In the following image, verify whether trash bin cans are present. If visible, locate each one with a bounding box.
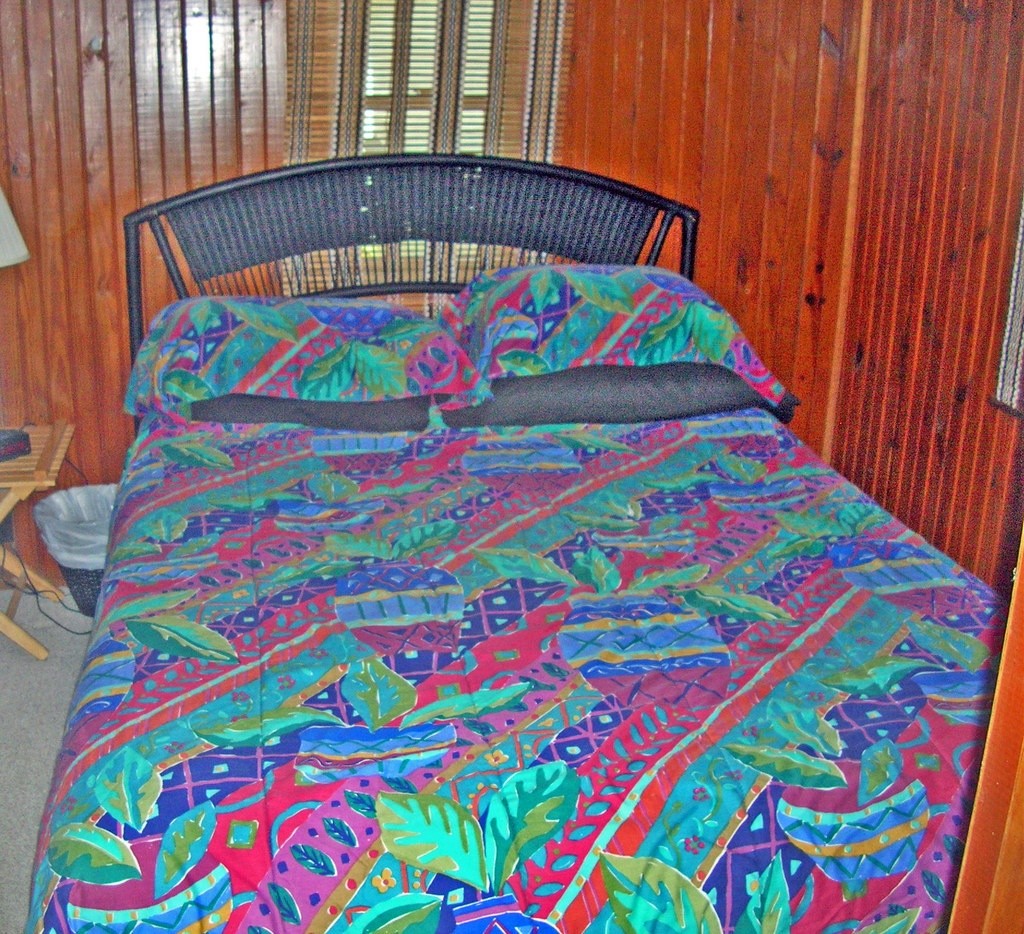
[34,484,119,616]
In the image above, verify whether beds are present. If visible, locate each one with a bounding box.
[25,152,1007,934]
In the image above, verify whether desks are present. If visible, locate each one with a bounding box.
[0,425,79,662]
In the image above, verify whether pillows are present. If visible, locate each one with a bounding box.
[121,294,493,417]
[439,267,798,421]
